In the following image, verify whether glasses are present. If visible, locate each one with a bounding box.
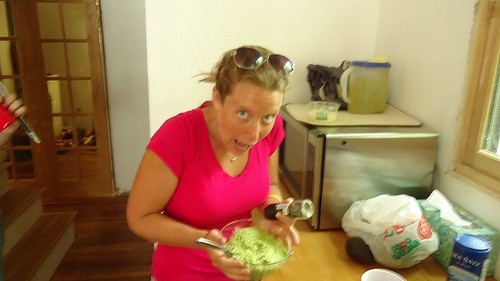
[230,47,295,78]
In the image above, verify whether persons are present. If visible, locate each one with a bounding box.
[0,90,26,146]
[127,46,299,281]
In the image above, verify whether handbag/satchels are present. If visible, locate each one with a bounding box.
[340,193,440,270]
[305,60,350,112]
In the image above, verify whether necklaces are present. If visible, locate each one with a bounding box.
[231,157,236,161]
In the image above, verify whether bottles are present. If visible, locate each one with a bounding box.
[258,198,314,221]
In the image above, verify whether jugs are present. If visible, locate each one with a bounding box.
[339,60,392,113]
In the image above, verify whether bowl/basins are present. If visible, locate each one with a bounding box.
[307,101,340,122]
[220,219,296,279]
[361,267,406,281]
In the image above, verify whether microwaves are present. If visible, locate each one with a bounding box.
[279,103,438,230]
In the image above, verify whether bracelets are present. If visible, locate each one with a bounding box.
[267,194,283,202]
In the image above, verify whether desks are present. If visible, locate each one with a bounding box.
[251,187,449,281]
